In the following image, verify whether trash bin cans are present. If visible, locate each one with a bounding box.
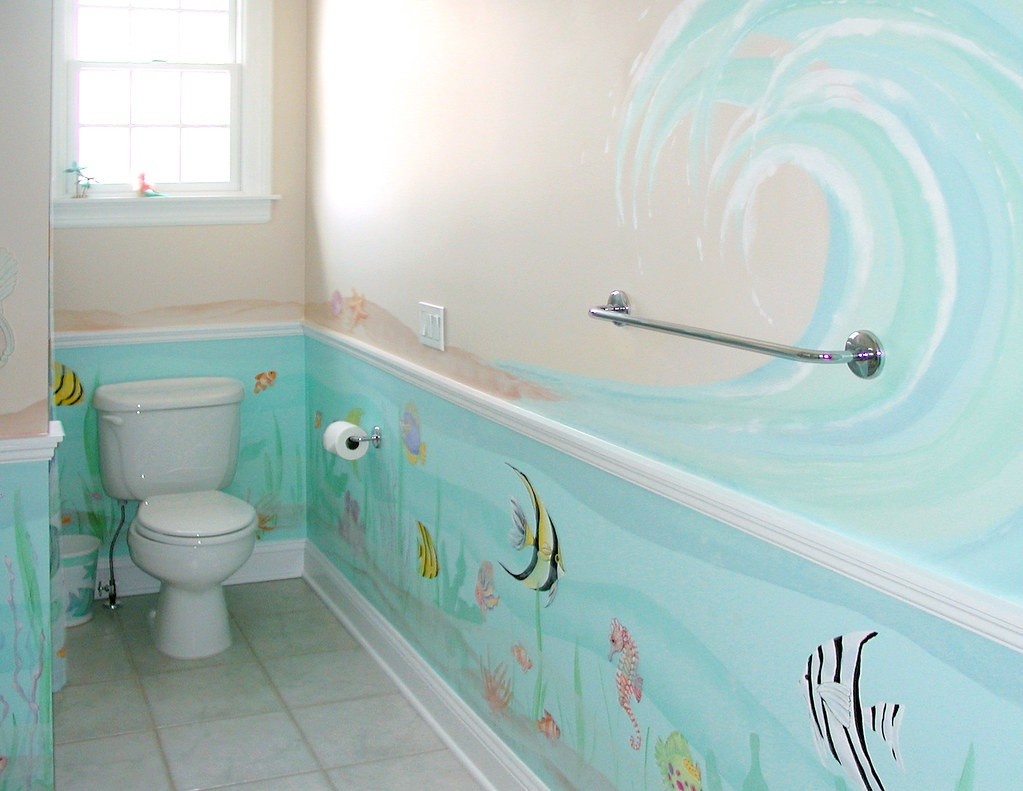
[59,534,101,628]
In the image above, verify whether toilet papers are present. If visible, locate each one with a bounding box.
[322,420,370,461]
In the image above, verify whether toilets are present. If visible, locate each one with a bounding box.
[91,374,262,662]
